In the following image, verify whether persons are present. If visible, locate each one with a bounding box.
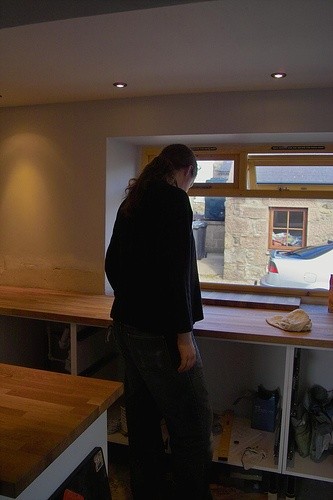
[105,144,214,500]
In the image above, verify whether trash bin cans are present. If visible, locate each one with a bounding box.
[191,220,208,261]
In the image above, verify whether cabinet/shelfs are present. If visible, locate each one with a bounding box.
[0,284,333,483]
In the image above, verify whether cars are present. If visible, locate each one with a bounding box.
[260,241,333,291]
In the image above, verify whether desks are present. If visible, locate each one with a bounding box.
[0,362,125,498]
